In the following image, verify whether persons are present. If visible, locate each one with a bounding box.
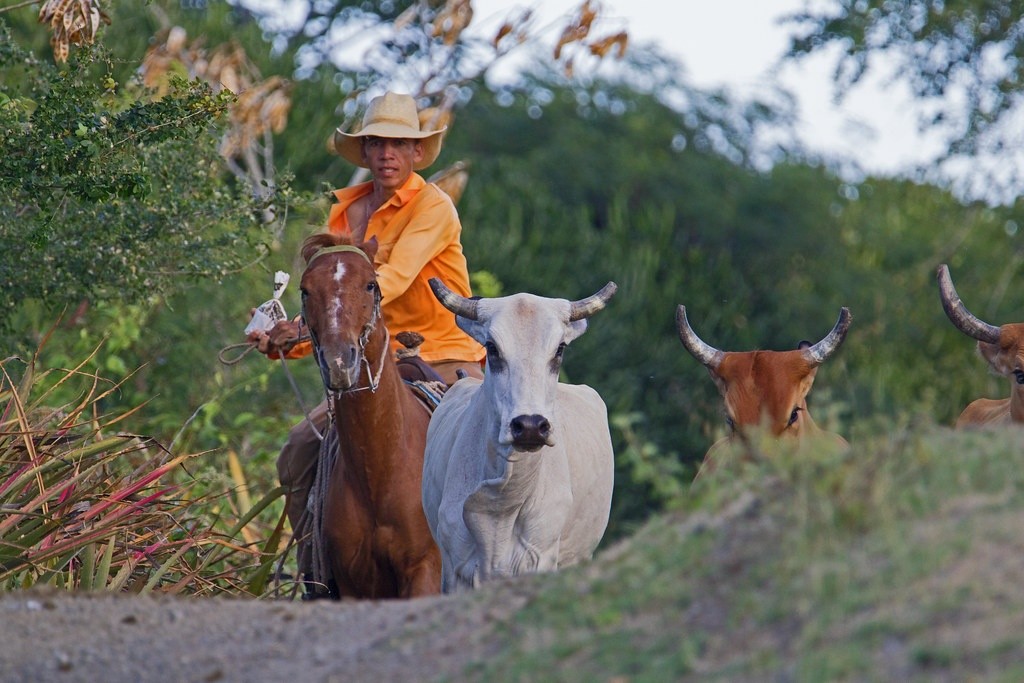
[240,90,498,603]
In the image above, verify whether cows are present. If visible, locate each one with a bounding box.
[936,264,1024,432]
[423,275,616,597]
[673,301,853,503]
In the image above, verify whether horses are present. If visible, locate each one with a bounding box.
[296,231,486,605]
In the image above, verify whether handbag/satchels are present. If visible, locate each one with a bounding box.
[244,270,291,335]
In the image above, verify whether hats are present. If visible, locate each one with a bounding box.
[335,90,444,171]
